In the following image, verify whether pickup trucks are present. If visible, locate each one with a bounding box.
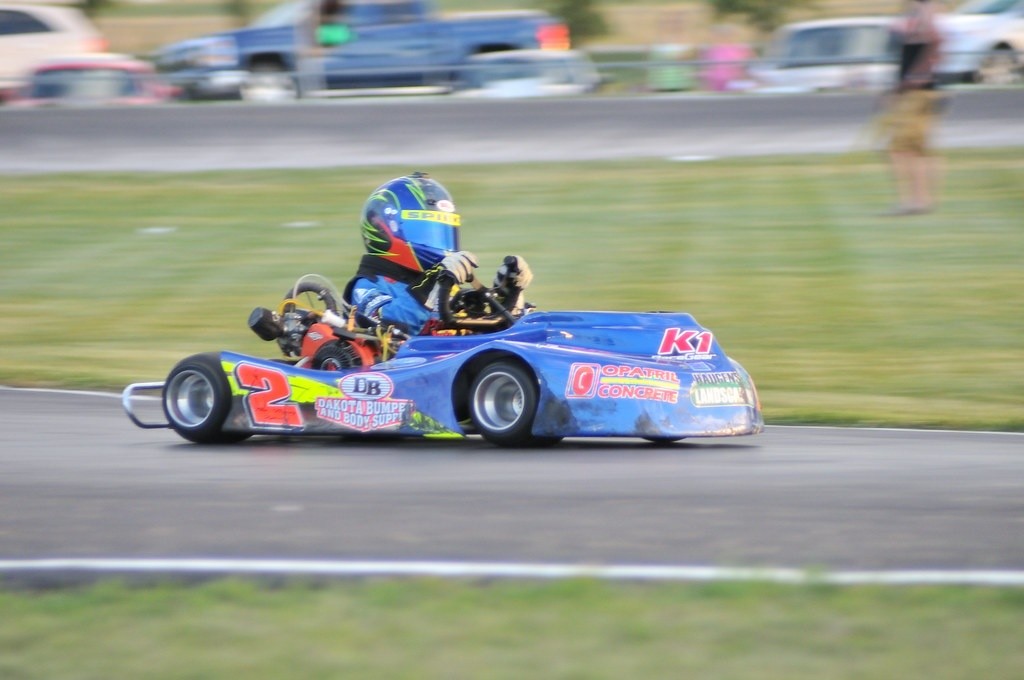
[151,0,573,100]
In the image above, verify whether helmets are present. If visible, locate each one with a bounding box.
[361,171,460,273]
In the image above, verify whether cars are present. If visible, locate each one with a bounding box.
[0,55,176,110]
[742,17,908,95]
[436,51,620,101]
[926,0,1024,88]
[0,1,107,90]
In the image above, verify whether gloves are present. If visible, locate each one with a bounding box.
[424,250,479,311]
[494,255,532,309]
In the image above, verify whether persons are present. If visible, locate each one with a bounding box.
[870,1,950,213]
[342,170,534,337]
